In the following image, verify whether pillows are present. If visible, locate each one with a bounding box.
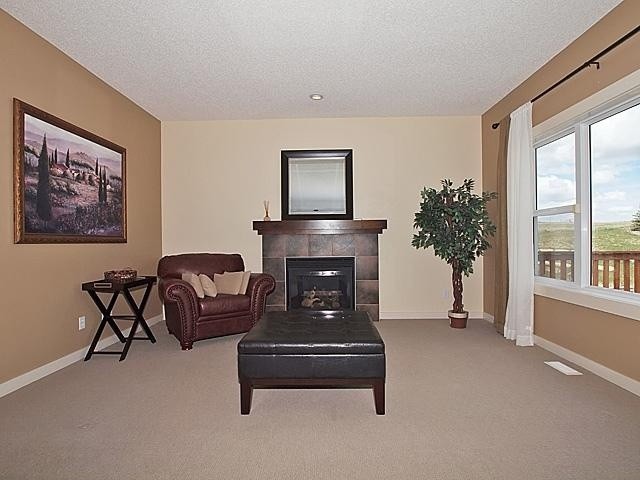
[181,270,251,298]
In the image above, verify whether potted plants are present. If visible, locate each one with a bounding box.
[412,177,498,330]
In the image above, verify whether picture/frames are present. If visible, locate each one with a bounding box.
[13,98,127,244]
[280,149,353,218]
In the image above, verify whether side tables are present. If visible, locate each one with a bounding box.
[81,275,157,361]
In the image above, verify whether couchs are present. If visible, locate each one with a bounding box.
[159,253,277,350]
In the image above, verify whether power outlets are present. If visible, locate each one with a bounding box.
[79,316,86,330]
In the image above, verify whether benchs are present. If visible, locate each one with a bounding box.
[236,310,386,415]
